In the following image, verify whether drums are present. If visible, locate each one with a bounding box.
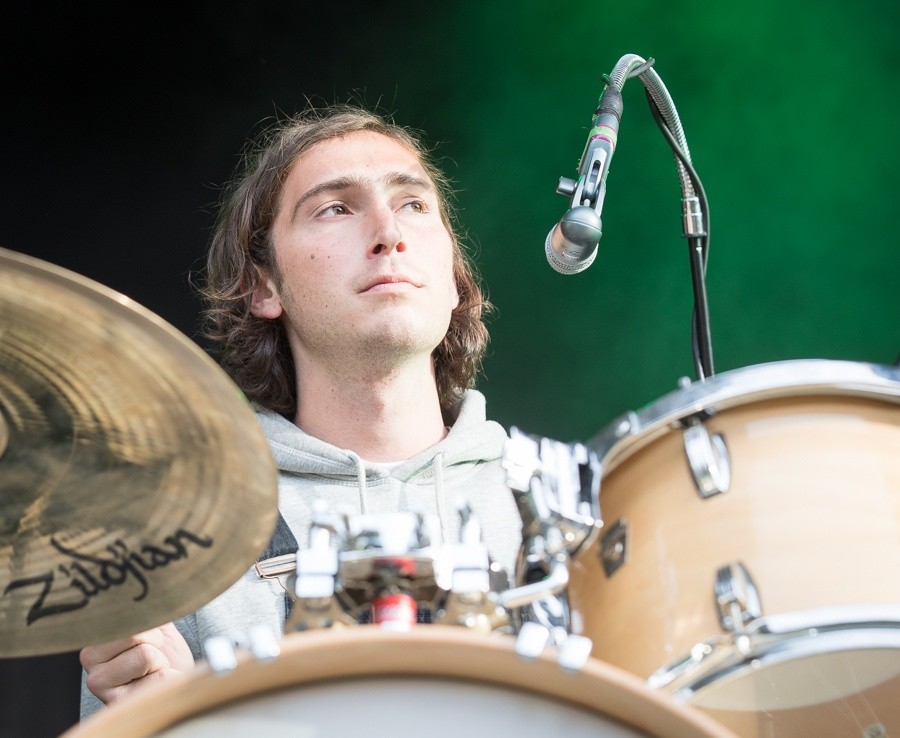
[55,620,739,738]
[514,359,900,738]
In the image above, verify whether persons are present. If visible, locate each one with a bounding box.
[79,108,638,738]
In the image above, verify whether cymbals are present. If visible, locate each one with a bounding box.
[2,247,281,659]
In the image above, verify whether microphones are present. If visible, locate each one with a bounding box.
[545,211,604,275]
